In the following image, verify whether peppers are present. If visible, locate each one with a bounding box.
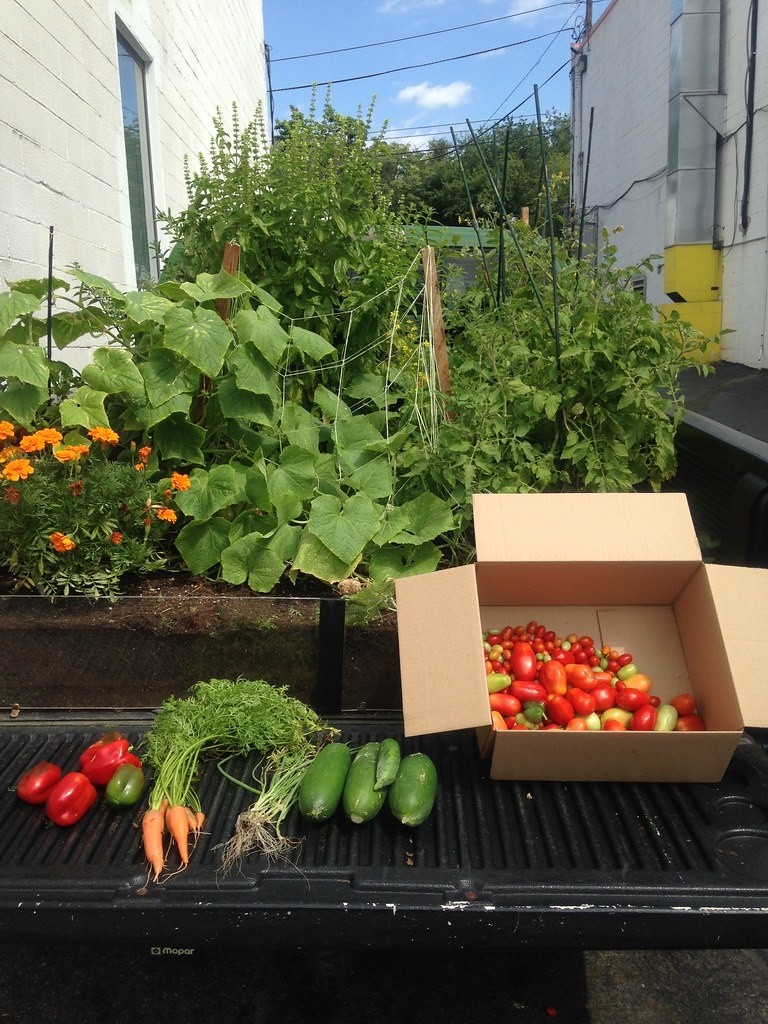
[16,731,145,827]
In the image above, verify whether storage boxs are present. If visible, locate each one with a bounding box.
[393,491,768,784]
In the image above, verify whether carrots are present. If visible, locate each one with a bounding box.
[135,674,341,894]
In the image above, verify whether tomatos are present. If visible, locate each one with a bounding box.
[482,620,705,731]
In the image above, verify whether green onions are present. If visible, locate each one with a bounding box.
[209,738,365,891]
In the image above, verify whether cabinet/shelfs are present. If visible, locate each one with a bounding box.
[0,721,768,1024]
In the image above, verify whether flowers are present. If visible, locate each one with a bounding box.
[0,416,192,603]
